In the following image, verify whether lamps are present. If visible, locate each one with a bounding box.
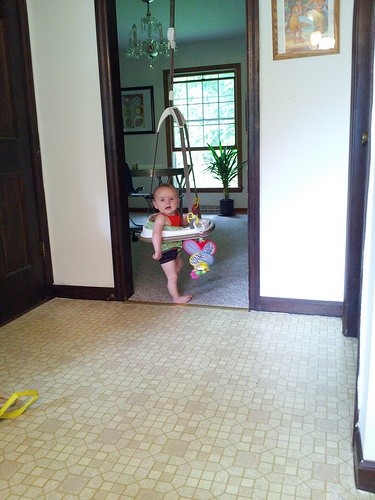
[124,0,178,70]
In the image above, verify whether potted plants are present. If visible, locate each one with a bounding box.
[203,141,247,217]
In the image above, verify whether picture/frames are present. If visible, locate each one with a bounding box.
[120,85,157,135]
[269,1,341,62]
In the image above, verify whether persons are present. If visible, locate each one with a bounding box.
[150,186,193,304]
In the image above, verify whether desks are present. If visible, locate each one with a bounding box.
[128,161,193,221]
